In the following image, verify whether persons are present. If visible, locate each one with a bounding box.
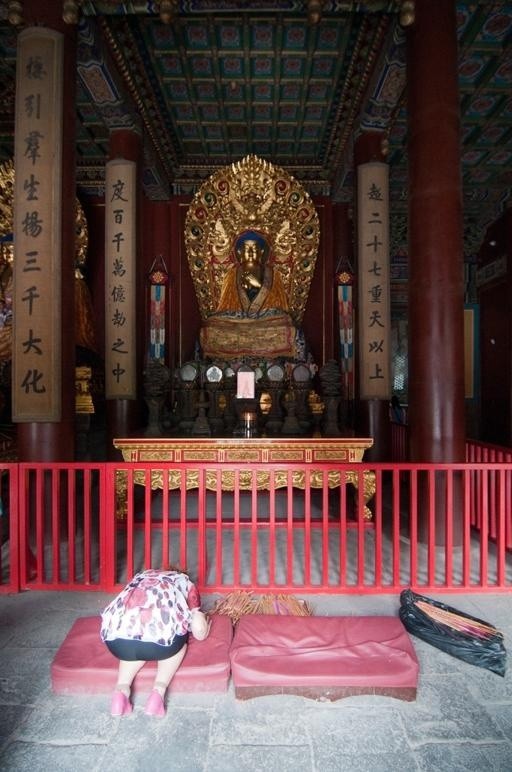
[214,232,288,316]
[101,567,212,717]
[0,234,14,327]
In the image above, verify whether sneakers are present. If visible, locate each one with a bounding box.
[111,692,132,716]
[143,689,165,717]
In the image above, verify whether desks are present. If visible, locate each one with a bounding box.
[112,437,379,522]
